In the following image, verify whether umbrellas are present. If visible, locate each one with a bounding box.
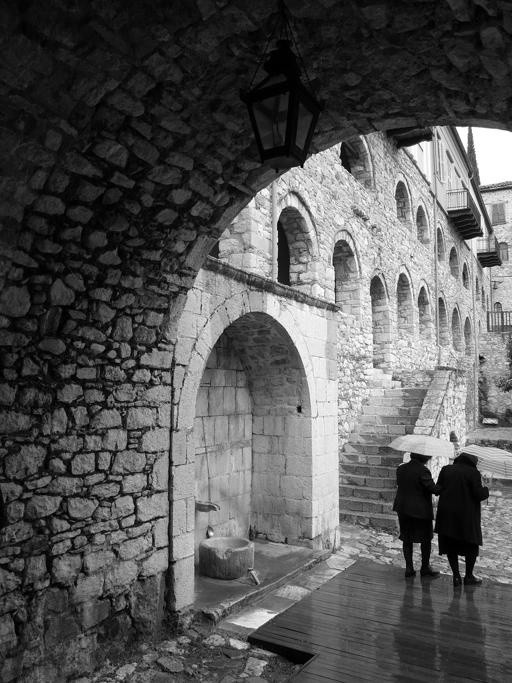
[454,443,512,481]
[384,432,457,460]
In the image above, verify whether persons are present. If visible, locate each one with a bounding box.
[391,452,439,580]
[433,452,491,588]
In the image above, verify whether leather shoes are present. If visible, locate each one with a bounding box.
[454,576,461,585]
[404,569,415,577]
[464,576,482,584]
[420,569,440,576]
